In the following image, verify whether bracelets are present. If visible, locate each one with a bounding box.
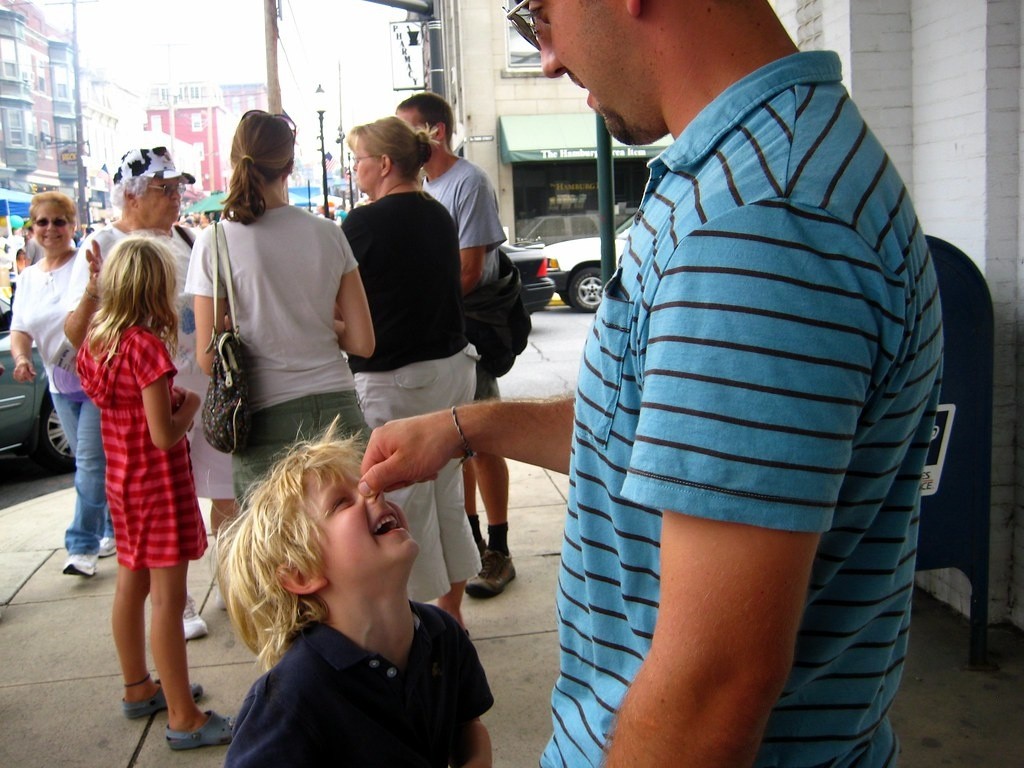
[82,286,101,304]
[12,352,33,368]
[452,406,474,471]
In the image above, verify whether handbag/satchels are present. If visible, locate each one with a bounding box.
[460,250,532,380]
[201,222,249,456]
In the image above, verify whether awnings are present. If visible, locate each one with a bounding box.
[498,114,674,164]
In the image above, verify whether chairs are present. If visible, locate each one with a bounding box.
[915,233,1000,672]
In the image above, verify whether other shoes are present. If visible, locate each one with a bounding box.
[181,598,206,640]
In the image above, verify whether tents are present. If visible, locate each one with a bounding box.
[0,189,33,284]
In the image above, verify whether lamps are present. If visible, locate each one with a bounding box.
[408,32,429,46]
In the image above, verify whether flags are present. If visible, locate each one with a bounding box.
[321,151,336,174]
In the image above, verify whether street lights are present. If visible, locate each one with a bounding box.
[314,84,332,218]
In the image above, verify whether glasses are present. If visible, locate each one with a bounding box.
[240,109,297,145]
[31,216,71,229]
[503,0,546,56]
[146,182,188,196]
[352,154,380,162]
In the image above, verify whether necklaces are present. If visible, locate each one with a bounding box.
[384,181,416,194]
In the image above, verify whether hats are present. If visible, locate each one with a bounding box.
[111,147,196,187]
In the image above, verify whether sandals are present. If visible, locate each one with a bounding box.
[164,708,237,749]
[119,678,203,720]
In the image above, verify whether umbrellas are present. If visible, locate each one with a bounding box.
[182,192,310,213]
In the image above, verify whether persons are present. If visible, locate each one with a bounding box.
[224,414,495,768]
[359,0,941,768]
[7,93,516,752]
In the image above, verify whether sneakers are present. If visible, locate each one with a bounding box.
[464,549,515,596]
[98,537,117,557]
[62,553,98,576]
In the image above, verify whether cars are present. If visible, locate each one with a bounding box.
[0,328,77,477]
[543,213,636,313]
[499,243,557,313]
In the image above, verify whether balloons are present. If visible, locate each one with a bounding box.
[9,215,24,228]
